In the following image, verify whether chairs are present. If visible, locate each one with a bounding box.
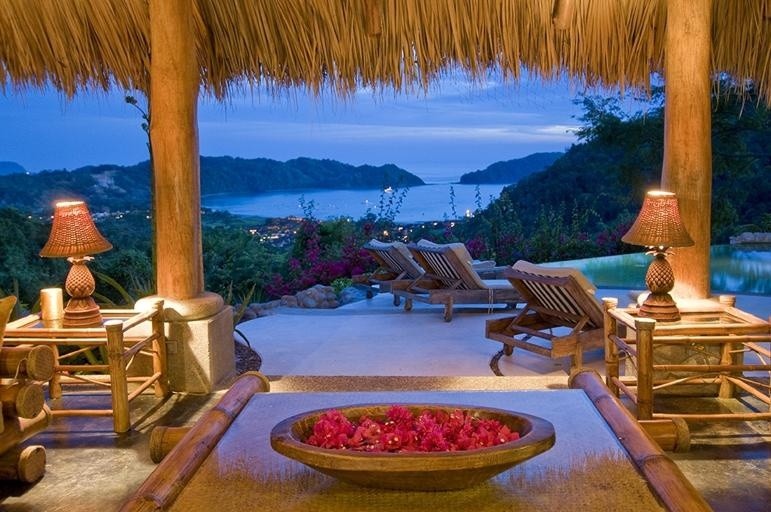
[484,258,627,376]
[362,239,528,323]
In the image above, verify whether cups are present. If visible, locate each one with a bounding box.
[40,287,64,321]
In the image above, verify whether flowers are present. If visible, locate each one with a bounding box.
[301,402,521,456]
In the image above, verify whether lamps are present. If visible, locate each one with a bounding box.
[40,201,114,328]
[621,189,696,322]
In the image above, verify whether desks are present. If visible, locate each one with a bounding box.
[3,298,172,434]
[120,369,712,511]
[602,295,771,422]
